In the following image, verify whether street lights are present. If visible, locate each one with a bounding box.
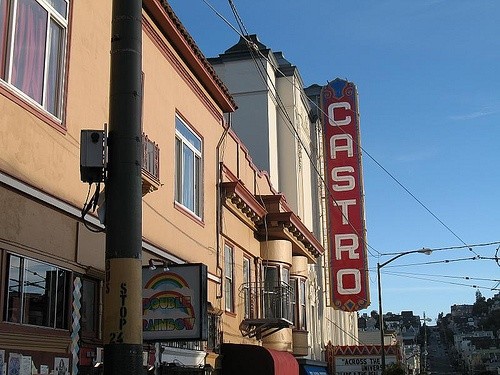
[377,249,432,375]
